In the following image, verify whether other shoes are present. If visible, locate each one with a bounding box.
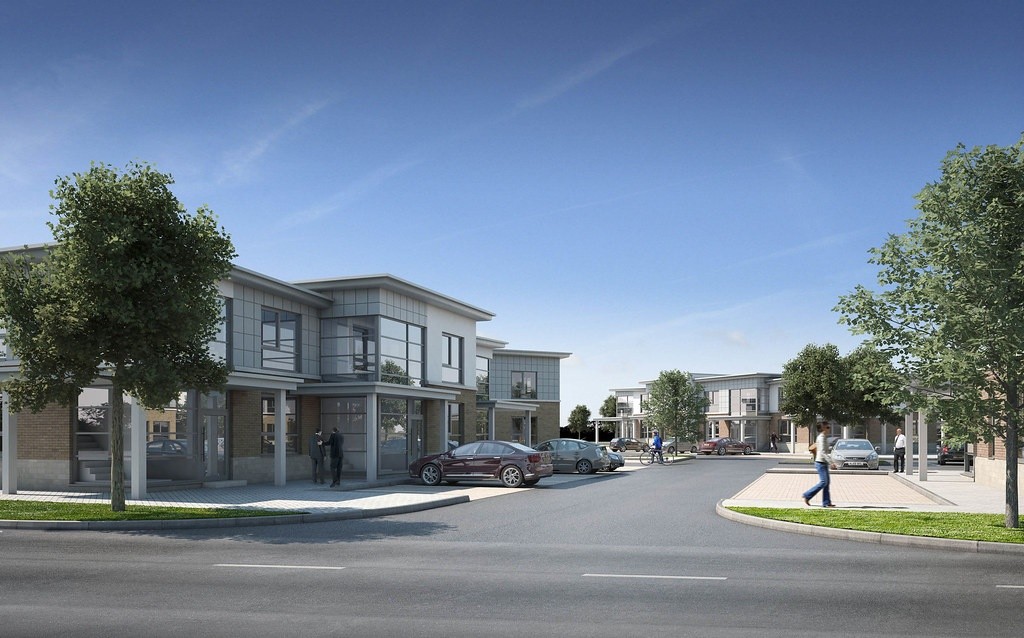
[894,470,898,473]
[900,470,904,472]
[801,495,810,506]
[823,504,836,507]
[330,482,340,487]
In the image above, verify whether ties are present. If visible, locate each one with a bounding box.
[894,434,900,446]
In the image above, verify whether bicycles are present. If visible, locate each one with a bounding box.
[640,448,674,465]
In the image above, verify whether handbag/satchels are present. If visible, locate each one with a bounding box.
[809,443,816,460]
[771,442,774,447]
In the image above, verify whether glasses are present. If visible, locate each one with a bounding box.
[823,426,830,429]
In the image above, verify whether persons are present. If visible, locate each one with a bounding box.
[309,430,327,484]
[768,432,781,452]
[648,432,664,464]
[317,427,344,487]
[892,428,906,472]
[801,421,836,508]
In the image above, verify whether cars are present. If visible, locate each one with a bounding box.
[606,451,624,471]
[408,441,554,488]
[661,436,699,454]
[218,438,274,452]
[144,439,208,457]
[936,444,973,466]
[531,438,610,475]
[829,439,881,470]
[381,436,459,452]
[825,436,842,454]
[609,437,650,453]
[700,437,754,455]
